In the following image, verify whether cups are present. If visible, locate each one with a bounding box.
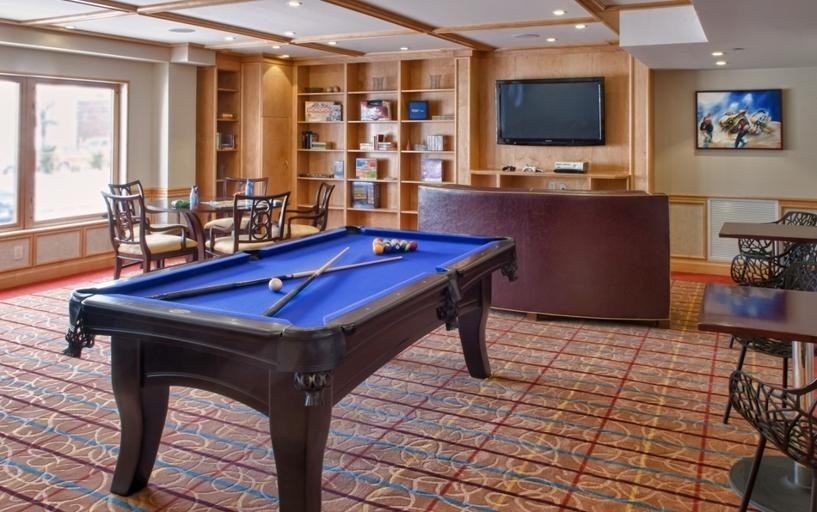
[372,77,384,91]
[429,75,441,90]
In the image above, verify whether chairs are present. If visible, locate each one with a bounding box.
[696,212,816,511]
[102,174,333,278]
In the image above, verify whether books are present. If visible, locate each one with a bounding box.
[301,135,448,150]
[218,113,236,150]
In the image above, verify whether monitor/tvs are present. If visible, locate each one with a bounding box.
[495,76,606,148]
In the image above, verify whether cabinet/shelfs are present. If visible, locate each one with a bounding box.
[242,53,299,223]
[197,54,241,225]
[295,50,458,234]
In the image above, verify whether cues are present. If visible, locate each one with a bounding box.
[144,247,404,315]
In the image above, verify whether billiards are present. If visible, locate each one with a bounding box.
[270,278,282,290]
[373,238,416,254]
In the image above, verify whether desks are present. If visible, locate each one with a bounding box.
[468,169,631,190]
[413,182,672,327]
[64,225,516,512]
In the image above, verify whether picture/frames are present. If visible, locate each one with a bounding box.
[694,87,783,154]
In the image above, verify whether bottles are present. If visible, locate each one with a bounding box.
[189,186,199,209]
[245,178,253,196]
